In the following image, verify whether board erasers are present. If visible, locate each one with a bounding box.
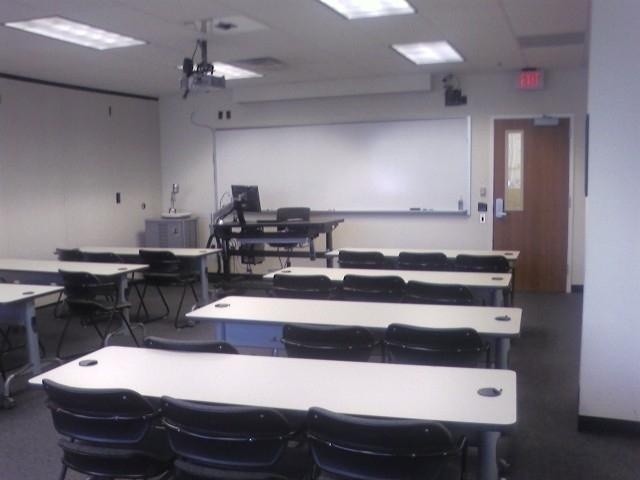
[410,208,420,210]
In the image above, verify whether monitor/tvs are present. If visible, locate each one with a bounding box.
[230,185,261,213]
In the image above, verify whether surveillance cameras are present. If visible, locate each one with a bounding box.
[441,74,455,84]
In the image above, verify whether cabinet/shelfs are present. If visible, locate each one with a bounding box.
[143,215,197,249]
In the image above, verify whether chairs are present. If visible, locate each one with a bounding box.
[40,378,164,480]
[0,248,525,406]
[152,393,296,478]
[303,403,464,479]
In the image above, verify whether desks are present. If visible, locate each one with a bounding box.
[29,345,519,477]
[209,218,345,272]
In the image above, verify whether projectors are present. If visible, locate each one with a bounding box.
[178,72,225,95]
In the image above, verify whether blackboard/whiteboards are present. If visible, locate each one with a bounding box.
[212,115,471,216]
